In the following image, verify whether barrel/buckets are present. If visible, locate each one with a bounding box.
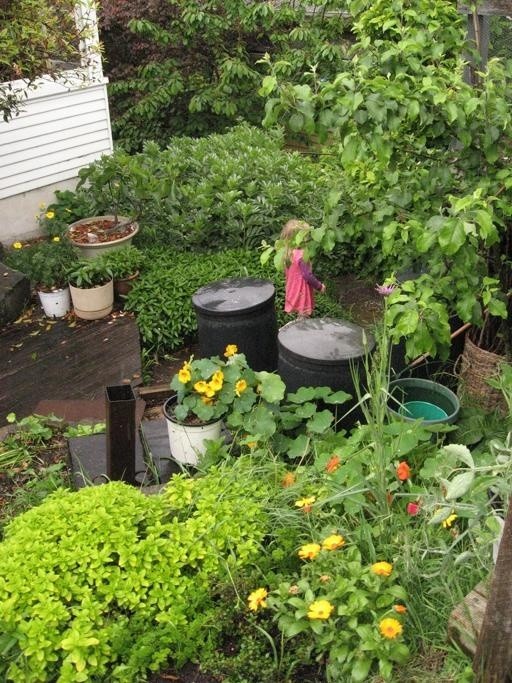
[398,401,448,420]
[69,279,114,320]
[385,378,460,426]
[37,288,72,318]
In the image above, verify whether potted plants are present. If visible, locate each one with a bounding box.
[13,172,162,320]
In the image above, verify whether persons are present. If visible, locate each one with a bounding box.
[277,219,326,319]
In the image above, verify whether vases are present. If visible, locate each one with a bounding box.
[383,378,461,447]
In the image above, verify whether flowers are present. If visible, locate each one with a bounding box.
[172,343,259,427]
